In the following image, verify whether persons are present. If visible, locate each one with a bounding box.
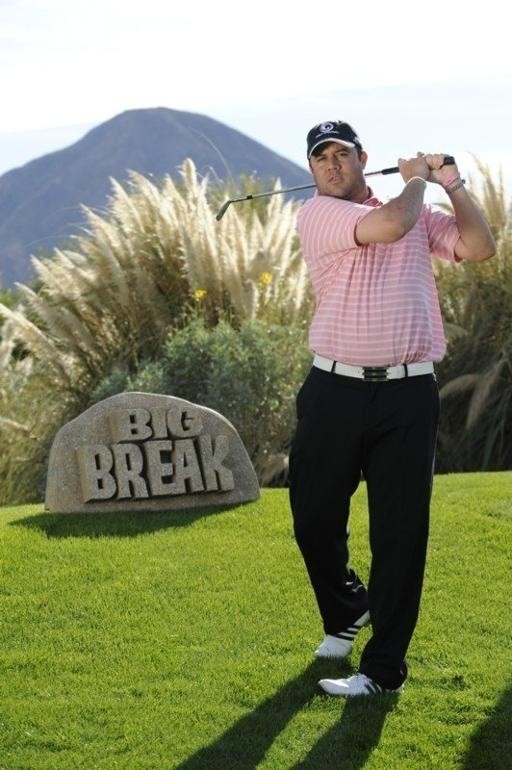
[288,118,499,699]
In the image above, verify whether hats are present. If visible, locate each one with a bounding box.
[307,120,358,159]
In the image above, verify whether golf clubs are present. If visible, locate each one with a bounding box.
[216,158,454,221]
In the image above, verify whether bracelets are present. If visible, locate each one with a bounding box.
[446,179,467,195]
[441,170,460,188]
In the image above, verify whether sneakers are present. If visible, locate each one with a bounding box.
[318,673,395,697]
[314,609,370,659]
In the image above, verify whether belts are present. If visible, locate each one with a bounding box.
[312,354,435,382]
